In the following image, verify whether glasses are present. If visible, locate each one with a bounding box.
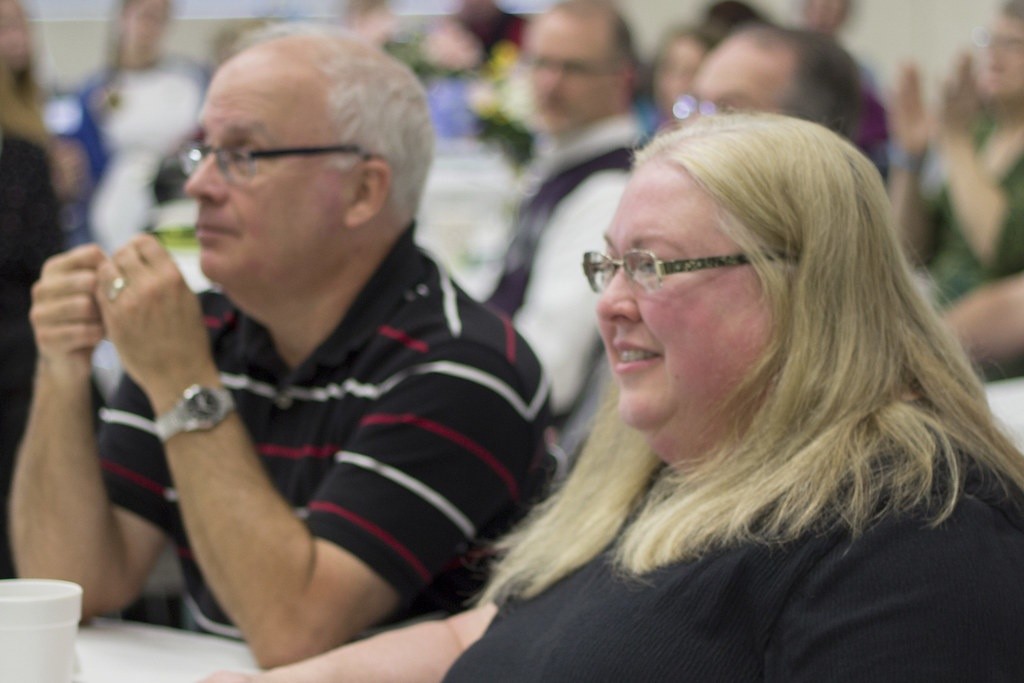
[672,93,724,119]
[177,141,371,187]
[581,251,787,293]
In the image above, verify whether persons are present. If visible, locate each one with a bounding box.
[9,32,570,672]
[0,2,1023,495]
[275,111,1023,682]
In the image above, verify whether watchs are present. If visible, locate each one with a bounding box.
[156,385,241,446]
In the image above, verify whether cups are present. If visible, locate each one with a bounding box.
[0,577,83,683]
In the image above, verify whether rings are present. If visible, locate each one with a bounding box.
[104,276,126,300]
